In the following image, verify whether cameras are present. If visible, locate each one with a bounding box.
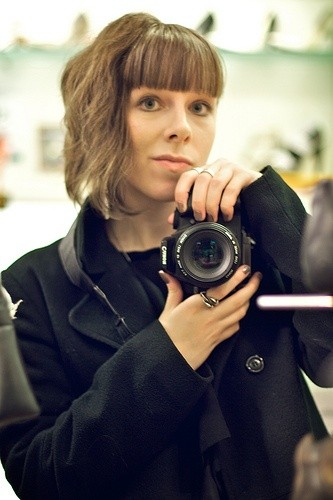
[160,194,252,296]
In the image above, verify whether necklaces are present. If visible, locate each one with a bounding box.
[106,221,130,262]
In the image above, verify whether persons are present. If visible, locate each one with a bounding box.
[0,12,333,499]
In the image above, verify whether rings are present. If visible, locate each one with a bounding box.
[200,291,219,308]
[192,167,215,177]
